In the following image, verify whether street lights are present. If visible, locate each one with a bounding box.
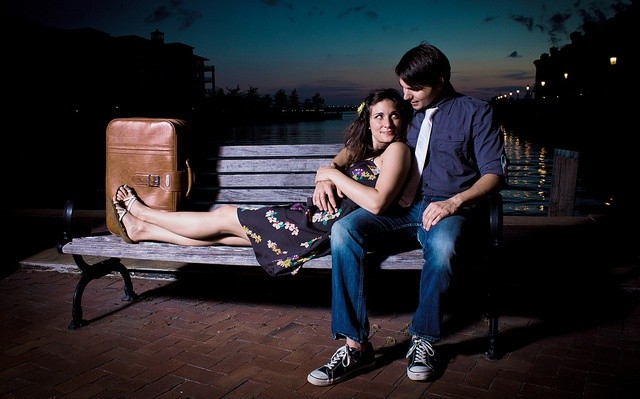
[541,81,545,95]
[526,84,530,97]
[610,56,617,91]
[509,91,512,99]
[564,73,568,95]
[517,88,519,98]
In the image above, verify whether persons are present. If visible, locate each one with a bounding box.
[307,44,511,387]
[110,88,410,275]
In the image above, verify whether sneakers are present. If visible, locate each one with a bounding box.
[406,334,436,382]
[306,341,378,386]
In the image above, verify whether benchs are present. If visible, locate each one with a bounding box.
[59,140,505,359]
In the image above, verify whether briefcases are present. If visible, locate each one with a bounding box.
[102,116,199,238]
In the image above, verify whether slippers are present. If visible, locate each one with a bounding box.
[107,196,135,244]
[114,184,139,209]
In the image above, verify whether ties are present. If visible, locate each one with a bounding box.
[396,105,439,208]
